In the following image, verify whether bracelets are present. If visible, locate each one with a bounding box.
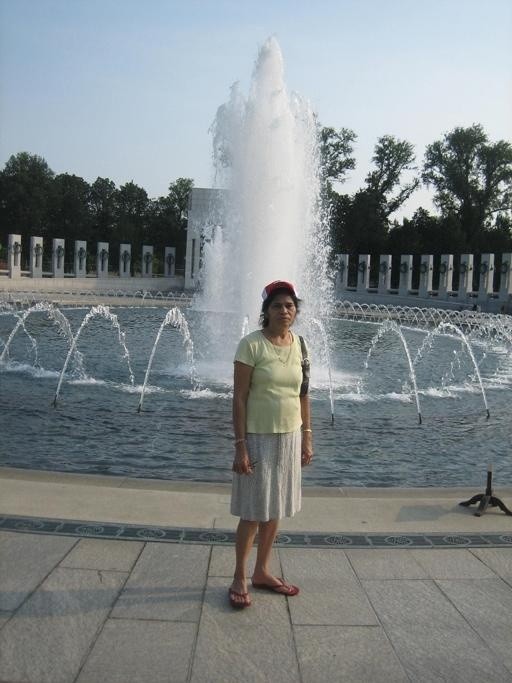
[235,439,248,446]
[304,429,312,432]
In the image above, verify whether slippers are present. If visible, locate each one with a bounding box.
[252,577,300,596]
[228,587,251,608]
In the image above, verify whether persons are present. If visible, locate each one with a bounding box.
[230,281,314,609]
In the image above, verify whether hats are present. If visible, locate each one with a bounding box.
[261,279,298,303]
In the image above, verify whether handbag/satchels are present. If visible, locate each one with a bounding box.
[299,358,311,397]
